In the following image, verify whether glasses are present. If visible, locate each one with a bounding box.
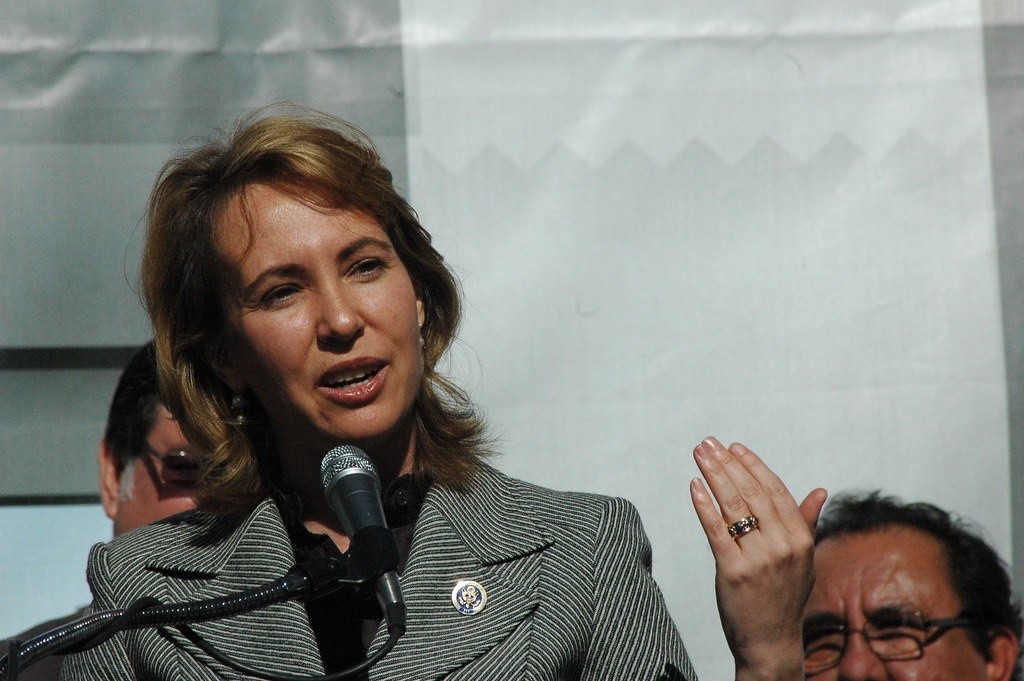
[802,609,979,669]
[123,431,200,486]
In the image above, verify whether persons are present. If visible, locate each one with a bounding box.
[60,101,827,681]
[803,494,1024,681]
[0,340,198,681]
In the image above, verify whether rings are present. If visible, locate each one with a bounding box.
[728,514,758,539]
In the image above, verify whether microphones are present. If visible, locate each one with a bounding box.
[321,445,407,635]
